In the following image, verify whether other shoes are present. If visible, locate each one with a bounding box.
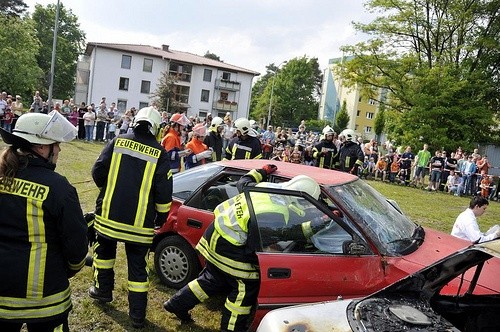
[164,299,195,322]
[129,312,144,327]
[88,286,112,301]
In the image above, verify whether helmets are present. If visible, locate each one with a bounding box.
[208,117,227,132]
[133,107,161,135]
[0,113,77,144]
[234,118,258,137]
[192,125,207,136]
[284,175,321,211]
[322,126,337,139]
[339,129,358,148]
[170,114,191,125]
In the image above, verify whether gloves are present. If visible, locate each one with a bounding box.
[323,210,341,221]
[263,164,277,175]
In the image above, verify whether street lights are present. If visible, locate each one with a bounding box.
[267,60,288,128]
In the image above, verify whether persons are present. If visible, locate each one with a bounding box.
[335,128,365,176]
[222,117,262,160]
[160,113,193,174]
[0,90,120,144]
[312,125,340,171]
[222,112,317,166]
[88,106,173,328]
[0,109,87,332]
[361,138,432,189]
[451,196,500,243]
[119,107,213,145]
[204,116,224,162]
[431,146,500,202]
[164,164,344,332]
[183,123,213,169]
[82,211,95,267]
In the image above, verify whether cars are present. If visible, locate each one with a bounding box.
[146,158,500,332]
[252,232,500,332]
